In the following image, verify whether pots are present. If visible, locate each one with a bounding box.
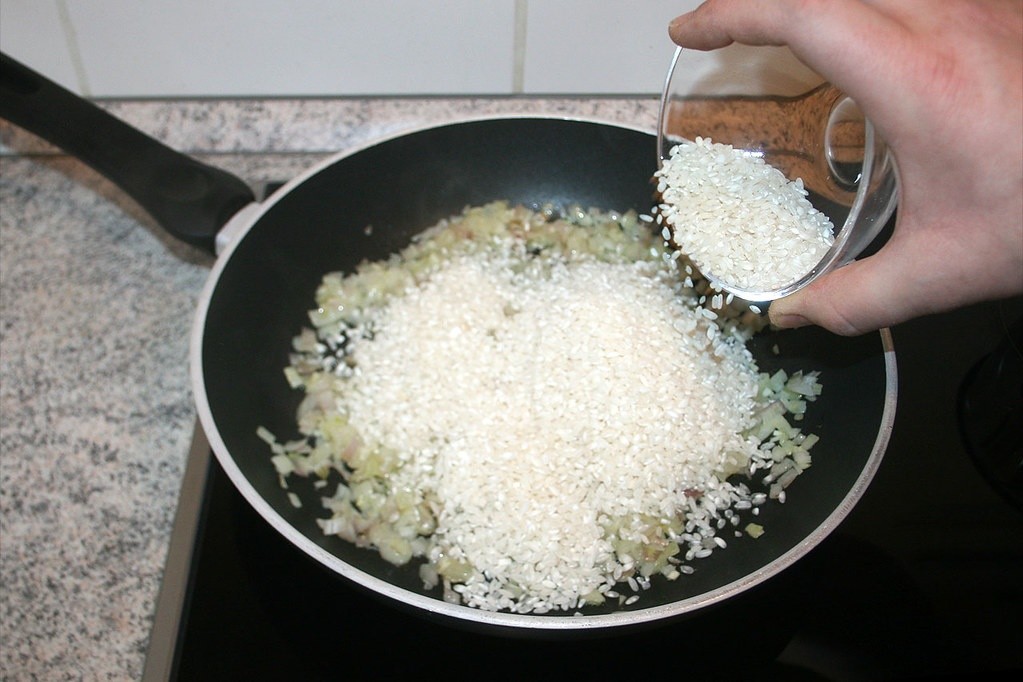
[0,46,897,633]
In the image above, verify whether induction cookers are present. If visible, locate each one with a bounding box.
[111,174,1020,682]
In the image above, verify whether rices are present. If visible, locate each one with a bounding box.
[339,137,835,615]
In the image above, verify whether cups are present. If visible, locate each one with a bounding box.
[656,31,903,302]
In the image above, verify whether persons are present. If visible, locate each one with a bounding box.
[668,1,1023,342]
